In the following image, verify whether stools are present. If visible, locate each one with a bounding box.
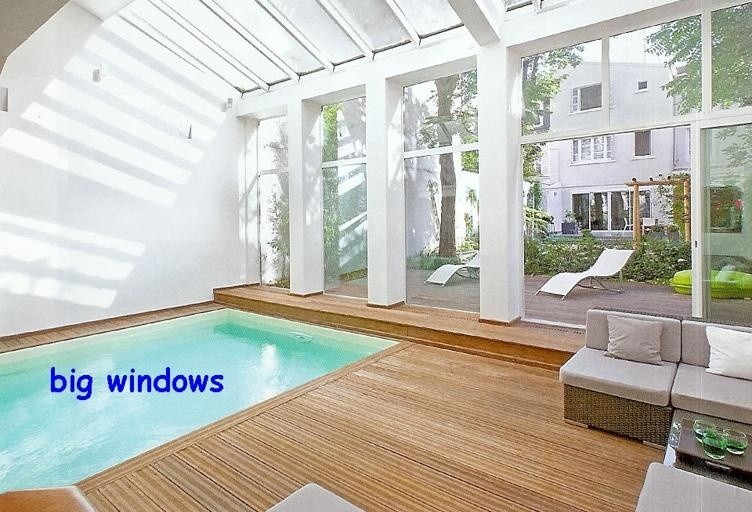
[633,462,751,512]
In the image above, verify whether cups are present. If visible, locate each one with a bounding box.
[692,419,748,460]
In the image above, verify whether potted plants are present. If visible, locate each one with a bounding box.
[560,209,578,234]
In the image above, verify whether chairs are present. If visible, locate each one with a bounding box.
[423,251,478,288]
[535,248,635,301]
[557,306,752,474]
[622,217,656,236]
